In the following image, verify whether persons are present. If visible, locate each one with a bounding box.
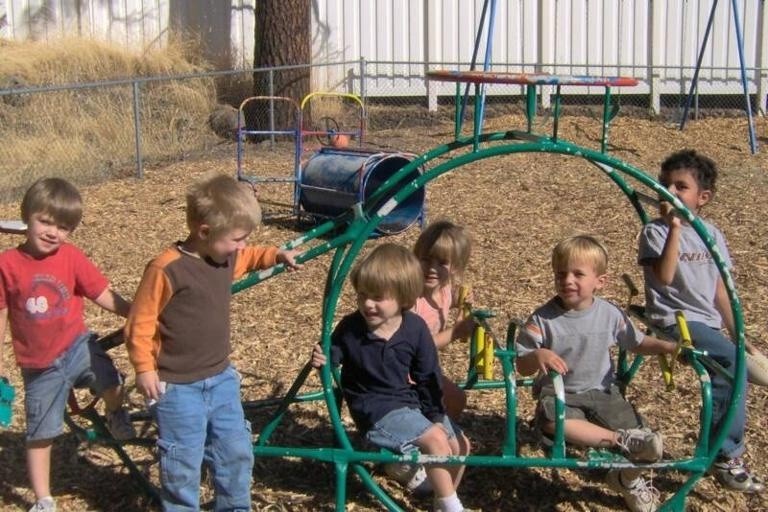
[639,147,768,490]
[312,240,478,512]
[516,233,695,511]
[2,175,133,511]
[405,219,481,426]
[123,167,304,512]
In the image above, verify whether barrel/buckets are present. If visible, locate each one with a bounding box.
[297,146,427,234]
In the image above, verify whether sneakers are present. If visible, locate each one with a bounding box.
[702,454,752,491]
[104,407,137,440]
[615,428,663,463]
[27,499,56,512]
[604,468,663,512]
